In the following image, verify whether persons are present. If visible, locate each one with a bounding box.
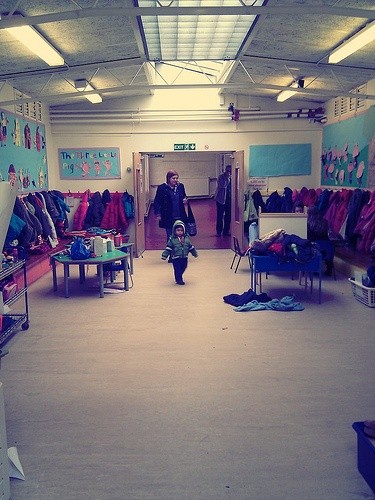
[161,220,199,285]
[154,169,189,263]
[214,165,232,237]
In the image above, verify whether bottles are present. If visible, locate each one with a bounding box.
[13,249,18,263]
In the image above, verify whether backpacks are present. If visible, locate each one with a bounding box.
[70,237,91,260]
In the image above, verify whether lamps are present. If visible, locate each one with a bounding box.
[75,80,103,105]
[2,13,65,66]
[277,79,304,103]
[326,20,375,65]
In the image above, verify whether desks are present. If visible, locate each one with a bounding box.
[113,242,134,278]
[251,252,324,304]
[54,249,128,298]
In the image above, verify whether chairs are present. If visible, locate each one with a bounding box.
[230,236,250,273]
[313,240,337,280]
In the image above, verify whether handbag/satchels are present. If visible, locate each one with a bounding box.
[3,281,17,302]
[186,219,197,237]
[249,239,266,254]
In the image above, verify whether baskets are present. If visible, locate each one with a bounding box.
[348,278,375,308]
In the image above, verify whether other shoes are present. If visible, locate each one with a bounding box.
[216,232,221,236]
[223,231,231,236]
[176,281,185,285]
